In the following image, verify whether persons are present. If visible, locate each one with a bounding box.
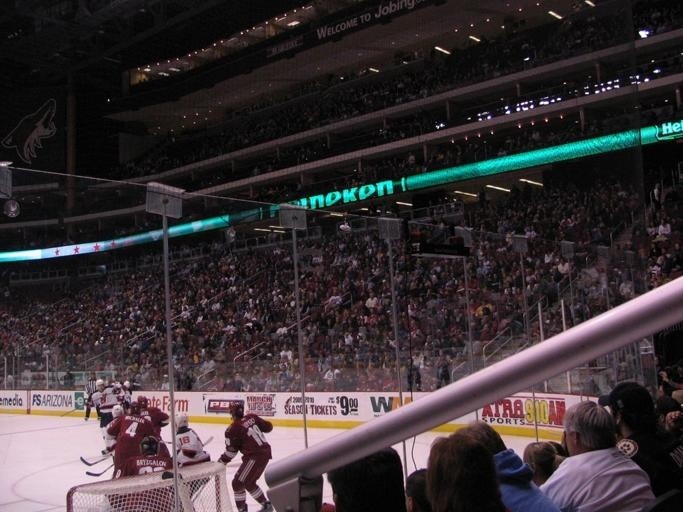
[0,3,681,390]
[326,390,683,512]
[84,389,274,511]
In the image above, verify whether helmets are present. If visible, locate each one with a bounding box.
[140,436,161,456]
[175,415,188,428]
[96,379,147,417]
[229,400,244,409]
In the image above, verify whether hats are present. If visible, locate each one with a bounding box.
[598,381,651,405]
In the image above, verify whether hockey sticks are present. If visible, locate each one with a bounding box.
[86,463,115,476]
[164,436,213,447]
[61,405,83,417]
[79,454,113,466]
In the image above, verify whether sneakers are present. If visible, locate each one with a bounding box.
[257,501,273,512]
